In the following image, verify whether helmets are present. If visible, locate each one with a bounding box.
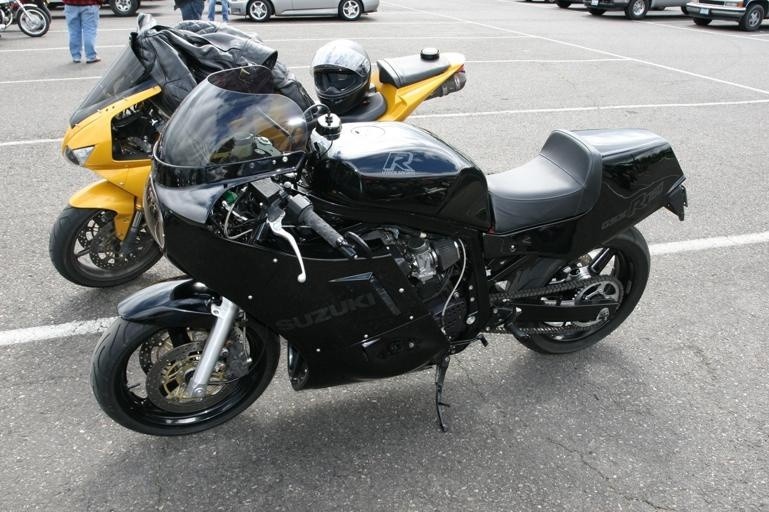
[309,40,371,114]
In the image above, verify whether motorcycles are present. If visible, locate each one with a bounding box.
[89,58,689,437]
[46,13,469,292]
[0,0,51,40]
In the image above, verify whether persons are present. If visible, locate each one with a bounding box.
[63,1,101,63]
[208,0,229,22]
[174,0,205,19]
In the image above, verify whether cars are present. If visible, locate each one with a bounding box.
[54,1,142,18]
[531,1,769,33]
[224,0,380,23]
